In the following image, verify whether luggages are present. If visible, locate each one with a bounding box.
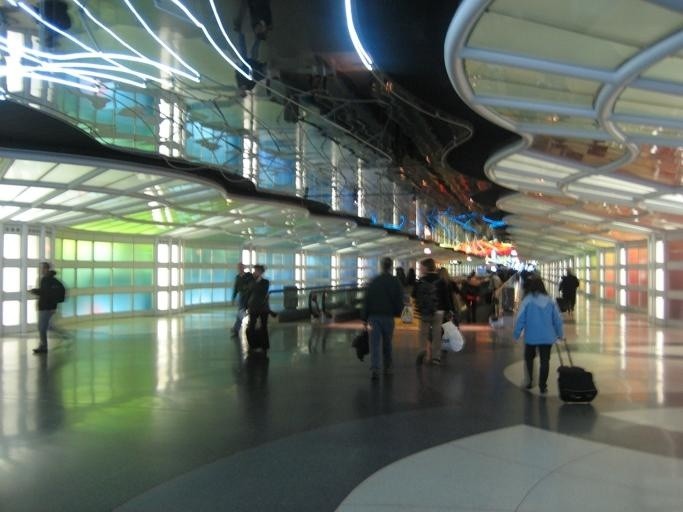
[246,329,269,348]
[555,338,598,403]
[556,290,567,312]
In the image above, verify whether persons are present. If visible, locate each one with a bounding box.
[556,267,578,315]
[360,256,406,382]
[241,263,270,350]
[393,264,512,326]
[23,260,73,353]
[509,273,565,394]
[226,261,251,337]
[409,256,446,367]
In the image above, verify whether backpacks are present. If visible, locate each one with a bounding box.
[414,279,441,316]
[52,275,65,304]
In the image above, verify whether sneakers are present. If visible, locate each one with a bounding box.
[33,348,47,353]
[416,350,427,366]
[433,359,440,365]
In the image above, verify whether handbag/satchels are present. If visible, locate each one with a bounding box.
[402,305,412,324]
[354,333,369,354]
